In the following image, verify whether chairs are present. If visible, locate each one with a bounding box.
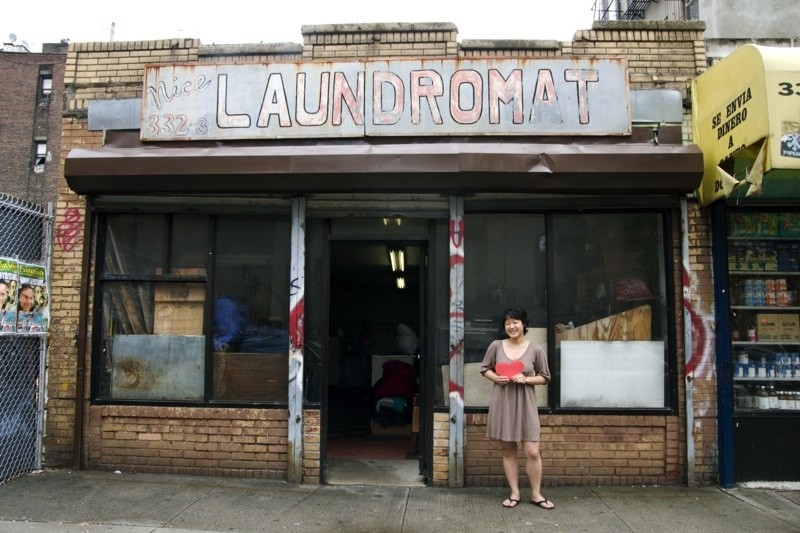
[372,355,415,439]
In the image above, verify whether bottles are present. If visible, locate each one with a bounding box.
[733,384,800,410]
[731,351,800,378]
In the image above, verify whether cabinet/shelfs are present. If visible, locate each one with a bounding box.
[728,236,800,381]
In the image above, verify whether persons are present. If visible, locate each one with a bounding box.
[0,279,47,333]
[480,306,555,510]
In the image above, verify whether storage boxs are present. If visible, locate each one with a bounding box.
[756,313,800,342]
[153,282,205,335]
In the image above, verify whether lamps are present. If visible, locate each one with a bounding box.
[387,244,405,289]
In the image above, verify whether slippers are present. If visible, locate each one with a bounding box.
[530,497,555,509]
[502,497,521,508]
